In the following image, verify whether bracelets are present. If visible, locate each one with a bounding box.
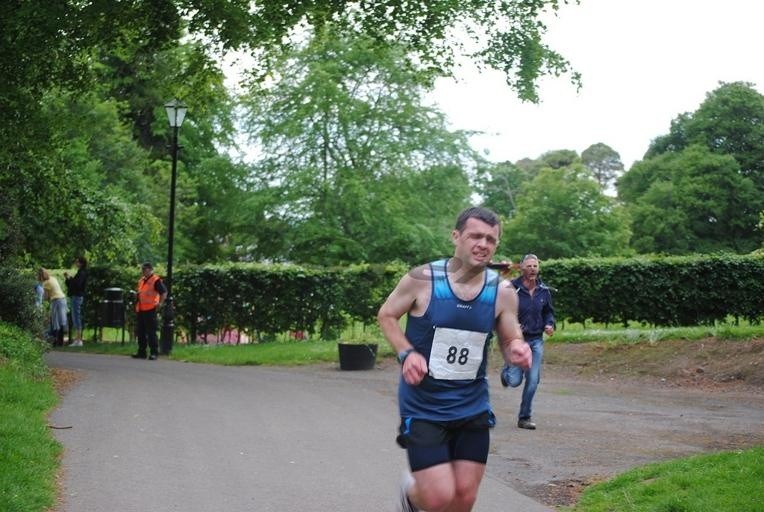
[395,347,415,365]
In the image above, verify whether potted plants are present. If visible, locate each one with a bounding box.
[337,332,378,370]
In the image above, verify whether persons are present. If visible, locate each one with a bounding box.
[61,255,87,347]
[129,263,168,361]
[500,252,555,431]
[376,204,533,511]
[36,268,68,346]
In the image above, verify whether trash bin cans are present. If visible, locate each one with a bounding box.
[98,287,125,345]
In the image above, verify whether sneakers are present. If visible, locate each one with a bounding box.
[149,355,157,360]
[400,476,419,512]
[500,370,509,387]
[131,353,147,359]
[518,419,537,431]
[68,340,83,347]
